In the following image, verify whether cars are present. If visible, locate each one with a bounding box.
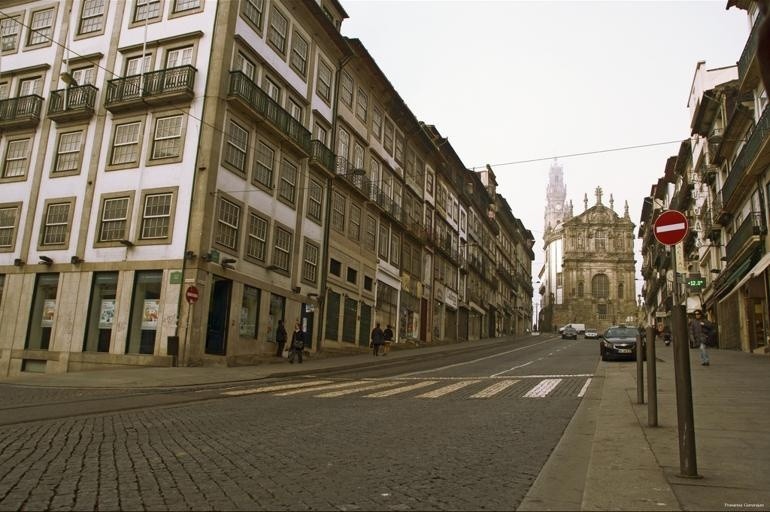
[562,327,577,340]
[584,328,599,340]
[601,324,647,362]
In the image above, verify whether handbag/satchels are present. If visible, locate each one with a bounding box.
[294,340,303,348]
[370,341,374,347]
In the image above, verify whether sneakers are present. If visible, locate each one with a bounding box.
[701,362,710,366]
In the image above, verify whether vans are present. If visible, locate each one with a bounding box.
[559,323,585,335]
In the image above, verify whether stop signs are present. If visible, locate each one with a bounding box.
[186,286,199,304]
[653,209,688,246]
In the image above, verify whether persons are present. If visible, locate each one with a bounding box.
[289,323,305,364]
[276,319,288,358]
[688,309,718,366]
[371,323,393,356]
